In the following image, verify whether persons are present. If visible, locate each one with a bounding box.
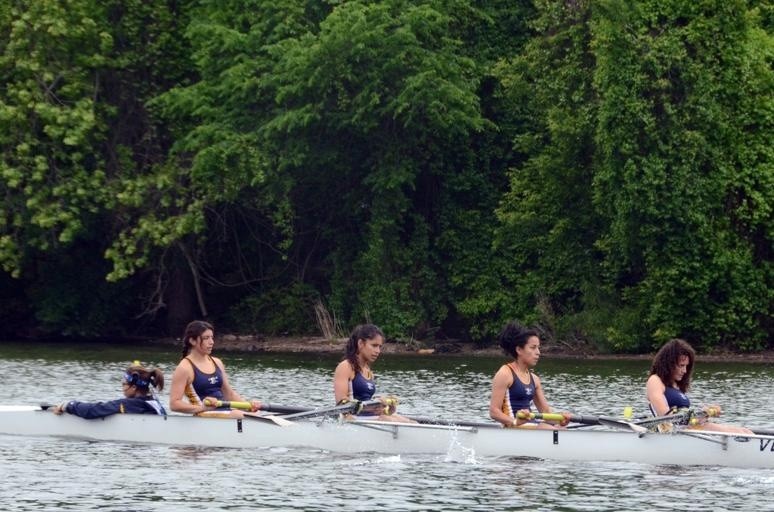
[646,338,755,435]
[488,321,571,430]
[169,321,261,418]
[334,325,419,424]
[47,365,167,420]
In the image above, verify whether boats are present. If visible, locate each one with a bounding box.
[0,399,774,469]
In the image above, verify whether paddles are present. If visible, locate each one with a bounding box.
[598,409,716,434]
[243,399,395,427]
